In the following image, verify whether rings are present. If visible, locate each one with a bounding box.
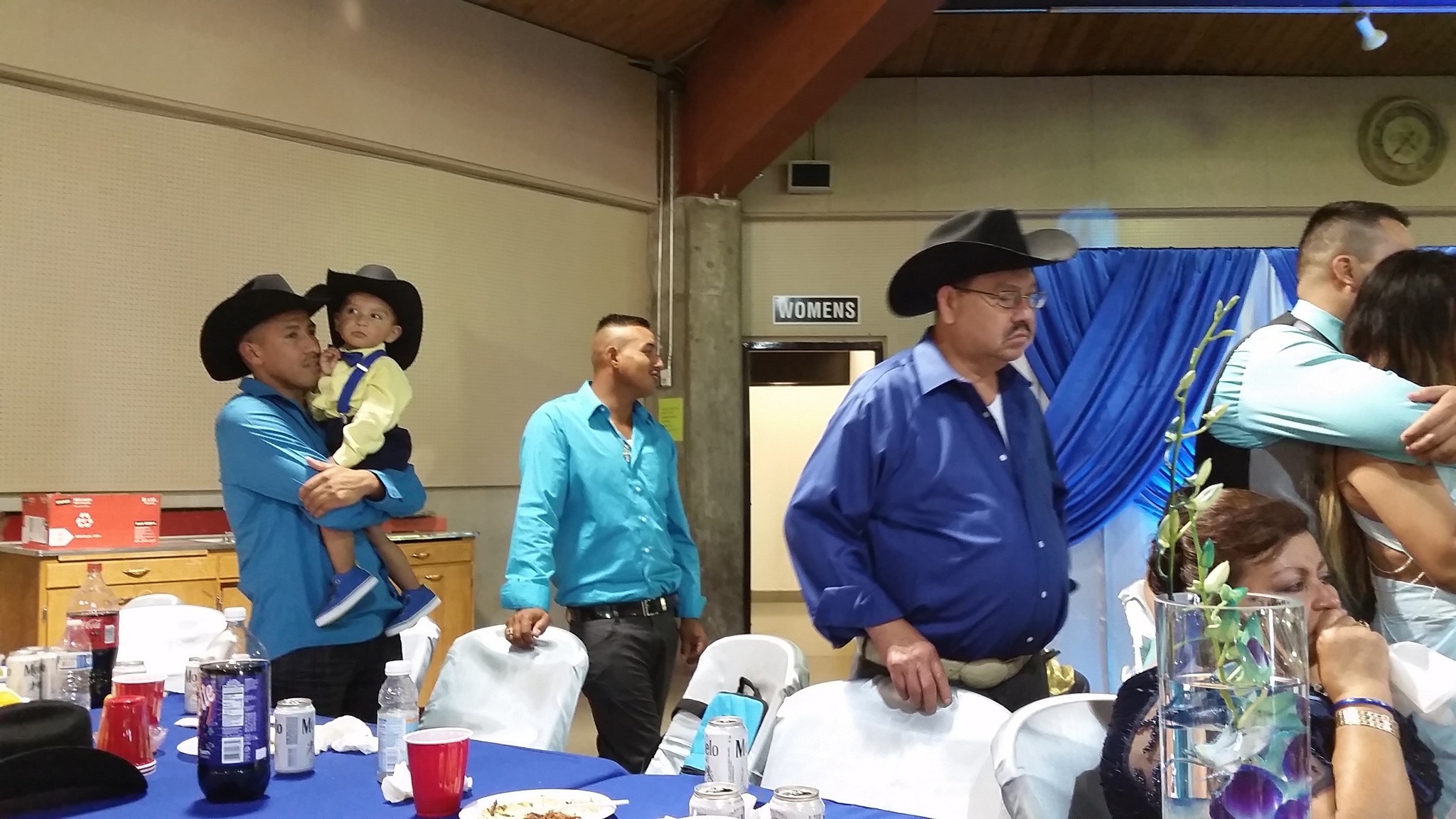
[504,628,514,635]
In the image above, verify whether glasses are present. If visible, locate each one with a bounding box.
[954,285,1049,309]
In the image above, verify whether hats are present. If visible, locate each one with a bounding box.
[323,264,423,371]
[885,207,1078,319]
[0,700,149,808]
[200,274,329,382]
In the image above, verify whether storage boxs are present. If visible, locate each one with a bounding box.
[14,484,165,551]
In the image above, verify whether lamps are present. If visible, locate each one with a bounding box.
[1354,4,1390,53]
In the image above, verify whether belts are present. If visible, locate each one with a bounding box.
[567,590,679,622]
[861,637,1035,688]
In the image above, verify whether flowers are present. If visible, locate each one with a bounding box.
[1144,299,1330,818]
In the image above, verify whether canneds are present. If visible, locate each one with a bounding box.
[5,644,56,704]
[273,696,317,774]
[186,656,208,715]
[684,720,824,818]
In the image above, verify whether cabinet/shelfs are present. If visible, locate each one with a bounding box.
[0,528,482,724]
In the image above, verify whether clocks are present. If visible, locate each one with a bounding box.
[1356,96,1450,186]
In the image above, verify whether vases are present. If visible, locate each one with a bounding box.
[1149,595,1314,819]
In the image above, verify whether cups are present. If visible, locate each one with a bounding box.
[4,648,68,702]
[403,727,473,817]
[92,668,169,776]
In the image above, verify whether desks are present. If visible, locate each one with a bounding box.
[0,651,945,817]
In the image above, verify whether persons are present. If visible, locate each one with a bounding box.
[1097,485,1444,819]
[1307,244,1455,819]
[1192,199,1454,625]
[782,208,1088,711]
[304,266,441,636]
[197,273,428,724]
[502,313,708,775]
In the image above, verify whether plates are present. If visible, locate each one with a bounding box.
[457,788,616,819]
[177,726,277,758]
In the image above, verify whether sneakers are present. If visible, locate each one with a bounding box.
[314,562,380,629]
[385,585,441,638]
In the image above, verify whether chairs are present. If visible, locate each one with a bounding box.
[412,607,1143,819]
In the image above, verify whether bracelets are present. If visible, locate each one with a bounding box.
[1331,699,1402,723]
[1334,708,1401,738]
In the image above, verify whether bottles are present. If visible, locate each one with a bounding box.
[378,658,421,786]
[67,562,122,708]
[194,607,272,805]
[55,618,93,714]
